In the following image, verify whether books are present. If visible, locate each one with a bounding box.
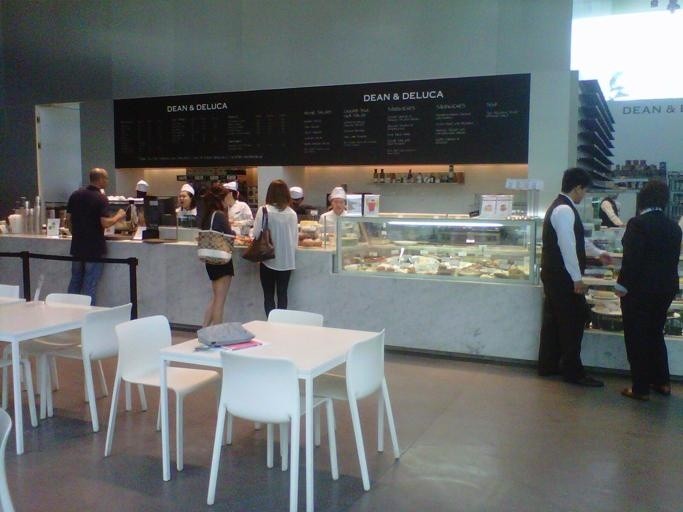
[221,340,263,351]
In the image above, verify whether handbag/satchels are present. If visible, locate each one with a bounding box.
[197,230,236,266]
[195,321,255,352]
[242,228,275,263]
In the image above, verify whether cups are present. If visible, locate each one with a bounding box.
[20,195,43,235]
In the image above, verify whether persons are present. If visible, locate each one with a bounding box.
[66,166,127,307]
[614,180,682,400]
[286,186,306,216]
[198,183,237,328]
[538,166,613,387]
[175,184,198,216]
[597,192,625,228]
[319,186,349,234]
[135,180,149,197]
[252,178,298,318]
[221,181,255,235]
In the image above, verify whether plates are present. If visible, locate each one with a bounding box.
[591,306,622,316]
[668,313,681,319]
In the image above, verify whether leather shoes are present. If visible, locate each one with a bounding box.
[571,373,604,387]
[621,387,649,400]
[654,384,671,395]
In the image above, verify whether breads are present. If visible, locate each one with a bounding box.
[344,251,529,280]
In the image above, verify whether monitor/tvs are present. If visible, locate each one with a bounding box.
[144,195,178,229]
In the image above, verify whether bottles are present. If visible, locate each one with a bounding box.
[615,159,649,192]
[372,164,454,183]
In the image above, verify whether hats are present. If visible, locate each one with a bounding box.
[328,187,346,201]
[136,180,148,192]
[181,184,194,196]
[289,187,304,199]
[223,182,237,191]
